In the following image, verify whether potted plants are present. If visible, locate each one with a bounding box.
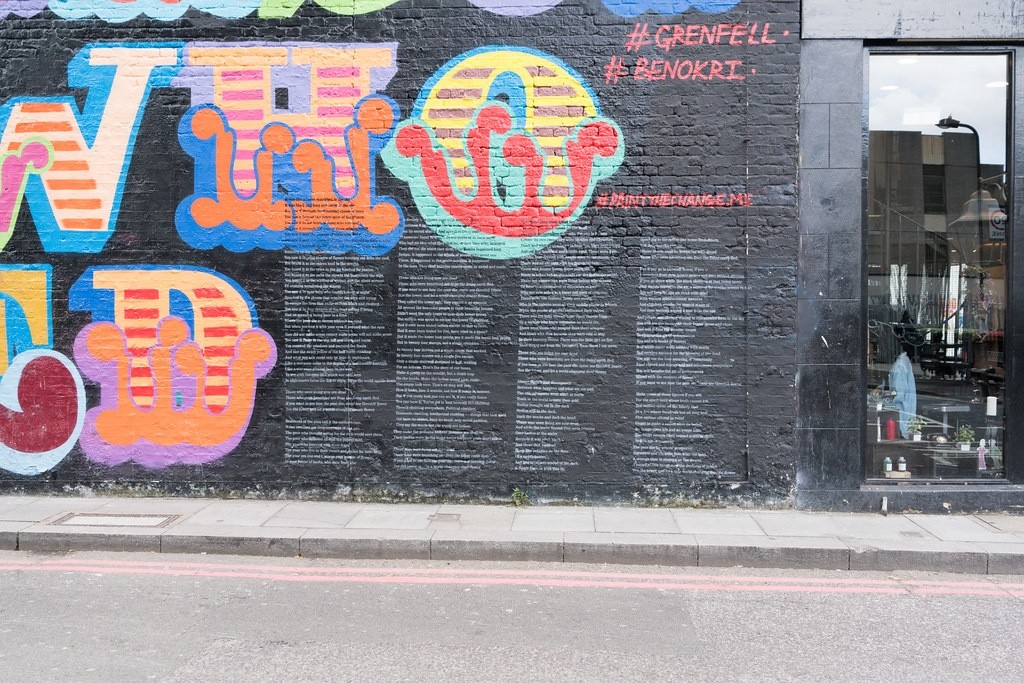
[868,389,973,451]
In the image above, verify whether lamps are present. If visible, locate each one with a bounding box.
[947,173,1006,267]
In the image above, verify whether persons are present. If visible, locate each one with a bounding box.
[896,310,917,362]
[888,351,918,442]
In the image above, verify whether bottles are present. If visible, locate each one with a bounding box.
[898,456,906,472]
[883,457,892,472]
[886,416,895,439]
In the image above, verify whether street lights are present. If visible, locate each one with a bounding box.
[934,114,985,369]
